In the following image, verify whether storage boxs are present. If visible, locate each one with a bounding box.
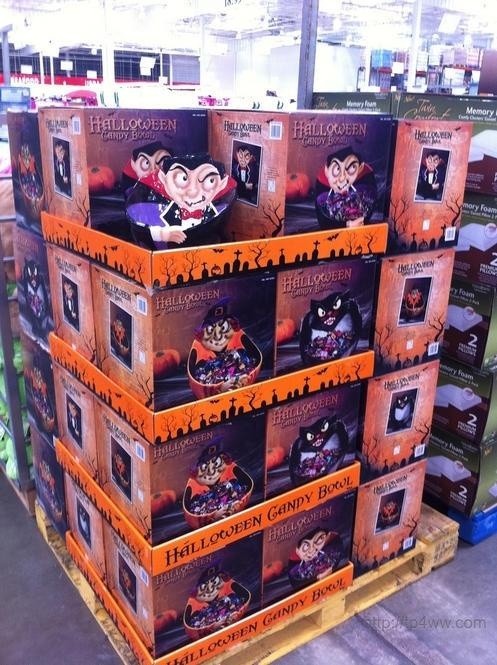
[0,93,494,664]
